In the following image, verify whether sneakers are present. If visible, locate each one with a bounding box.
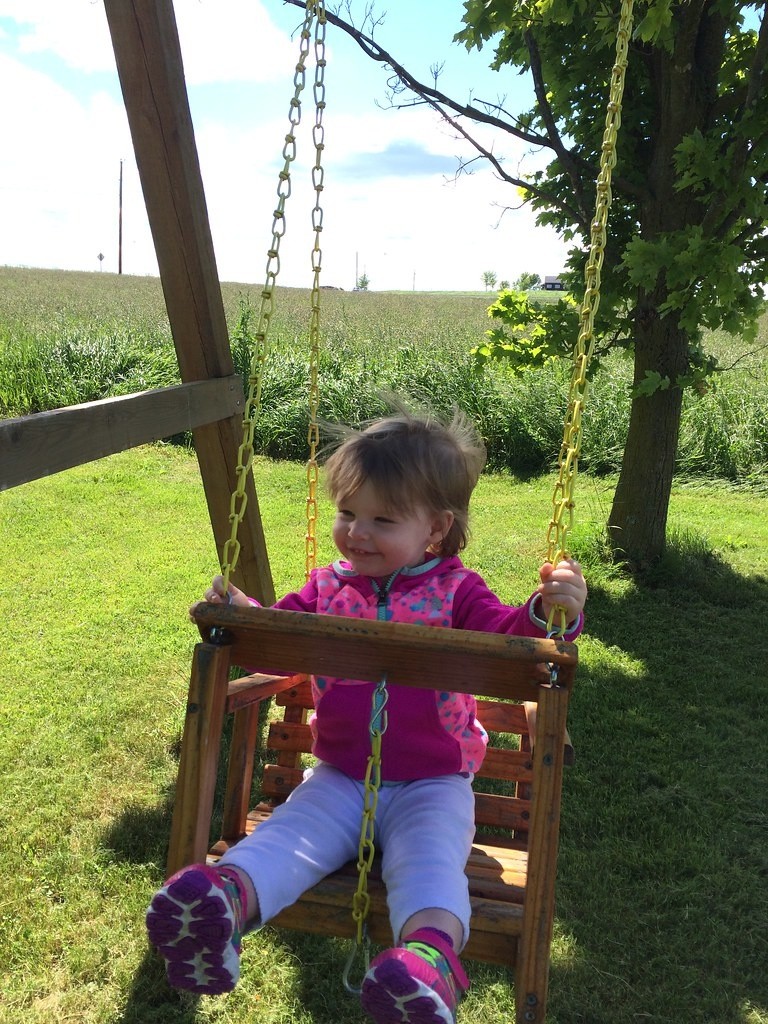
[361,927,469,1023]
[147,864,246,996]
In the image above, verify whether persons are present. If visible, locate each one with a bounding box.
[143,414,587,1024]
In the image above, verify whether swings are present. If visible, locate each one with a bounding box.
[168,0,634,1024]
